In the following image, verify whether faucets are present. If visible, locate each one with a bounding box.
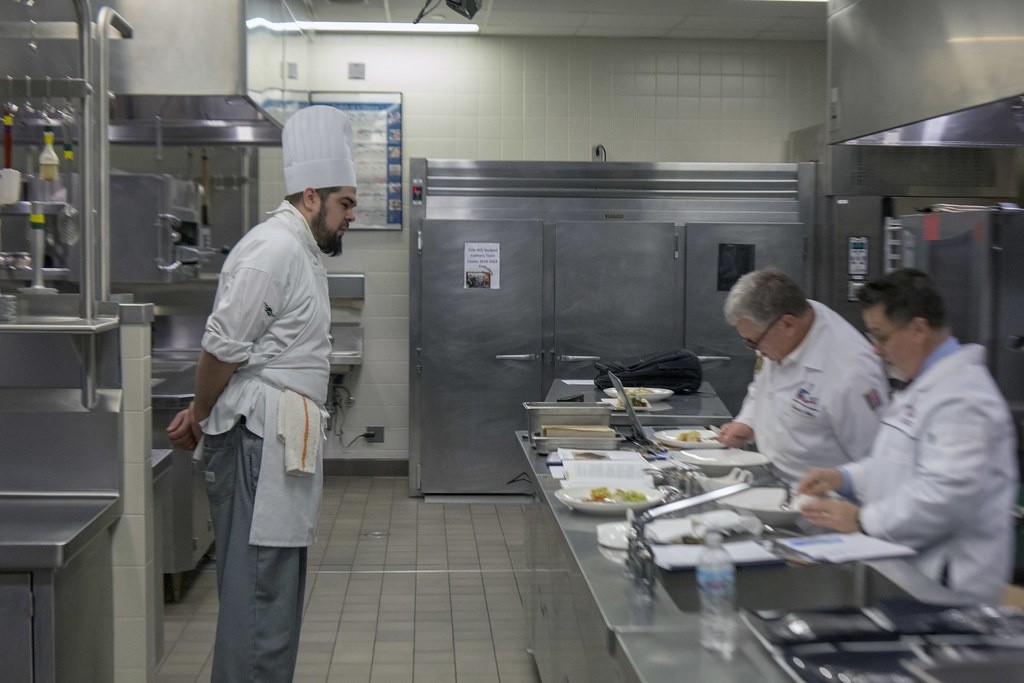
[641,476,794,518]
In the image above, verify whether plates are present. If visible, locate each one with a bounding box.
[672,448,772,474]
[654,429,728,448]
[555,487,668,516]
[604,387,674,401]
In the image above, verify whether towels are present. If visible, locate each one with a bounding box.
[276,389,320,478]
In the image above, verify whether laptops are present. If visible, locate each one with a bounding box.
[605,371,707,445]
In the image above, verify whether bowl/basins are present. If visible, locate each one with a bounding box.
[724,487,815,524]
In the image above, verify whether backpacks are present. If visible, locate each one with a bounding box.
[594,349,703,395]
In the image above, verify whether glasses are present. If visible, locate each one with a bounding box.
[861,317,914,347]
[738,313,783,350]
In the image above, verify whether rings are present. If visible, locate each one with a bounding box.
[821,511,829,519]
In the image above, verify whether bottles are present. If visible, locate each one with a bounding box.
[697,534,737,651]
[61,144,76,203]
[39,127,59,180]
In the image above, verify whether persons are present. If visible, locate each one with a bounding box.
[716,269,890,489]
[168,104,358,683]
[790,264,1019,608]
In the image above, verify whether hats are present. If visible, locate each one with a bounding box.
[282,105,357,196]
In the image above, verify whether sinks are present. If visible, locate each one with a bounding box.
[658,560,929,613]
